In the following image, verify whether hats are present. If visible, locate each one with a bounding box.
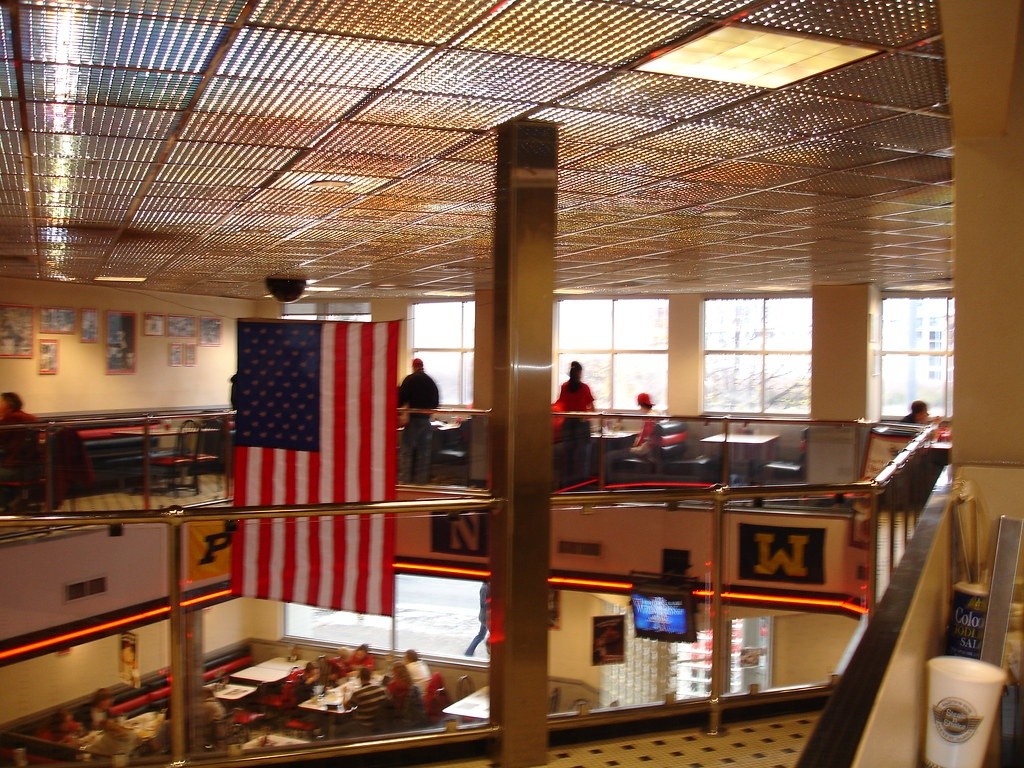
[413,359,423,366]
[638,394,655,406]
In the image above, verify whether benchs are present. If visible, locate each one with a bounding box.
[10,645,256,735]
[615,421,714,483]
[436,419,474,488]
[39,414,160,487]
[761,426,807,483]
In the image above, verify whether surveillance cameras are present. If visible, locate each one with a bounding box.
[266,278,306,304]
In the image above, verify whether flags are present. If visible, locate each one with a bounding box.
[229,317,402,616]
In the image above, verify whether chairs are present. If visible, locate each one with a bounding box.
[0,440,45,513]
[372,672,442,731]
[131,737,163,757]
[142,419,202,496]
[547,687,560,714]
[433,674,477,731]
[222,655,329,753]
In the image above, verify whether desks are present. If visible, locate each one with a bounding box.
[229,656,313,727]
[588,429,641,468]
[698,433,779,485]
[442,685,490,719]
[922,440,953,502]
[112,427,218,491]
[201,683,258,709]
[79,711,165,752]
[296,672,387,740]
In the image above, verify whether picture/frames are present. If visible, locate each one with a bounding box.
[105,311,138,375]
[169,342,197,367]
[0,303,35,359]
[38,339,59,374]
[199,315,224,347]
[79,307,98,343]
[143,312,166,336]
[167,314,198,339]
[39,306,75,334]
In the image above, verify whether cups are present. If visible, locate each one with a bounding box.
[924,656,1007,768]
[945,580,990,659]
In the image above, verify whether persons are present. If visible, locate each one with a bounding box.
[0,391,40,513]
[120,641,135,672]
[59,710,84,738]
[465,582,489,657]
[336,669,387,737]
[92,689,117,728]
[404,649,433,697]
[385,661,411,718]
[144,708,172,754]
[2,313,218,369]
[398,358,440,484]
[901,401,942,440]
[606,392,659,484]
[294,663,325,740]
[552,361,595,489]
[321,643,374,684]
[204,686,226,743]
[442,405,474,449]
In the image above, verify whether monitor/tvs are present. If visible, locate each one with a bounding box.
[631,594,698,644]
